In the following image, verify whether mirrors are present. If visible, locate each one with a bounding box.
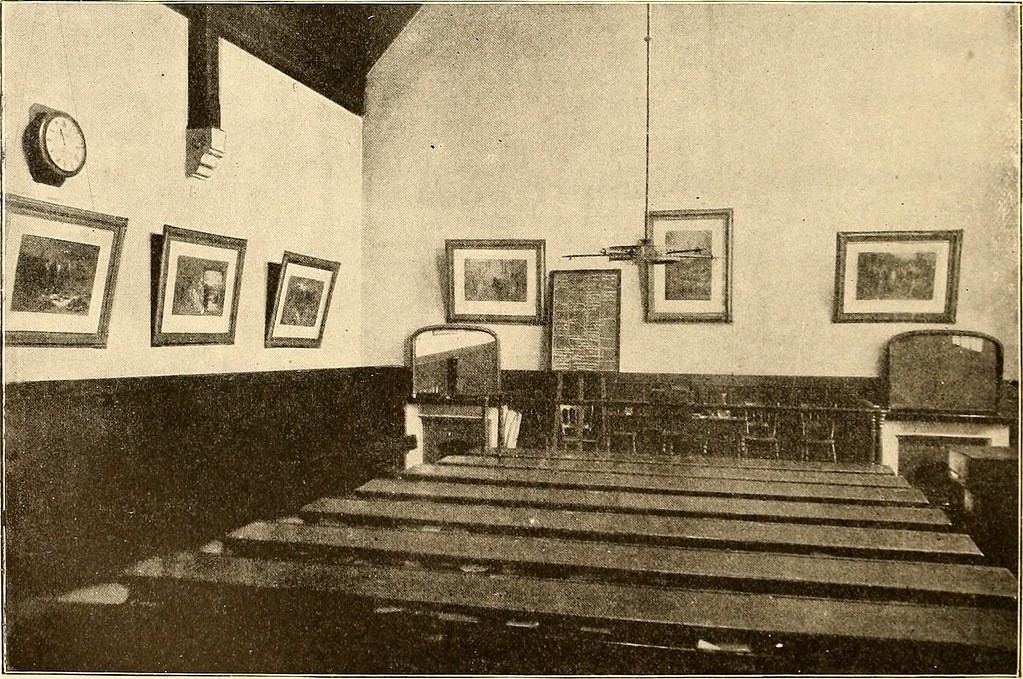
[411,324,500,404]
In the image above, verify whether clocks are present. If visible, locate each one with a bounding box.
[22,111,87,186]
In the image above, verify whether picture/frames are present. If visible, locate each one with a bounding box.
[446,238,545,326]
[266,252,339,347]
[151,224,247,346]
[836,228,960,324]
[645,210,732,322]
[5,193,128,348]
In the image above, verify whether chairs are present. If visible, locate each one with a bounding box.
[737,405,779,456]
[559,405,596,452]
[799,409,837,465]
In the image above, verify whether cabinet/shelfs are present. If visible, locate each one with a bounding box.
[869,405,1011,481]
[404,404,499,471]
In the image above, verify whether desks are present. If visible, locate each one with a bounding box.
[558,398,883,464]
